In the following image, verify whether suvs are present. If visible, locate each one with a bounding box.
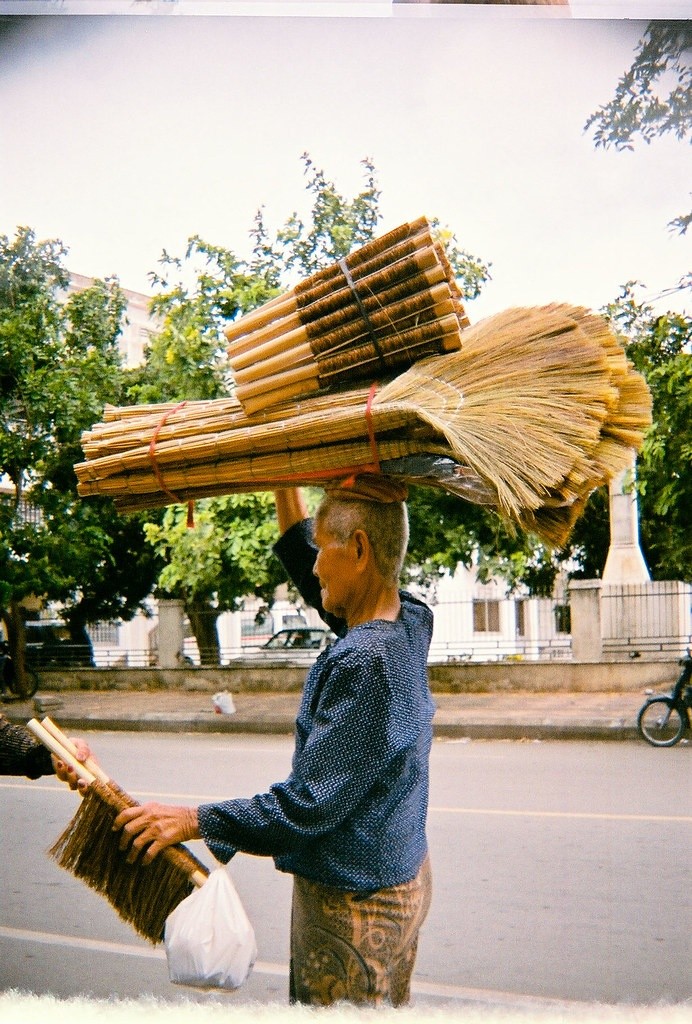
[23,618,98,667]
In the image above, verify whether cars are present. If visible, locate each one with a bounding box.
[227,626,339,667]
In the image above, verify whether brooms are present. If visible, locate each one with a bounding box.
[72,300,655,550]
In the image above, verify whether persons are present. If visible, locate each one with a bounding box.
[1,711,100,797]
[111,473,437,1012]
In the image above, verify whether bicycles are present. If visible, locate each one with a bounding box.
[0,641,41,703]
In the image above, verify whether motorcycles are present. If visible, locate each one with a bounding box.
[637,646,692,750]
